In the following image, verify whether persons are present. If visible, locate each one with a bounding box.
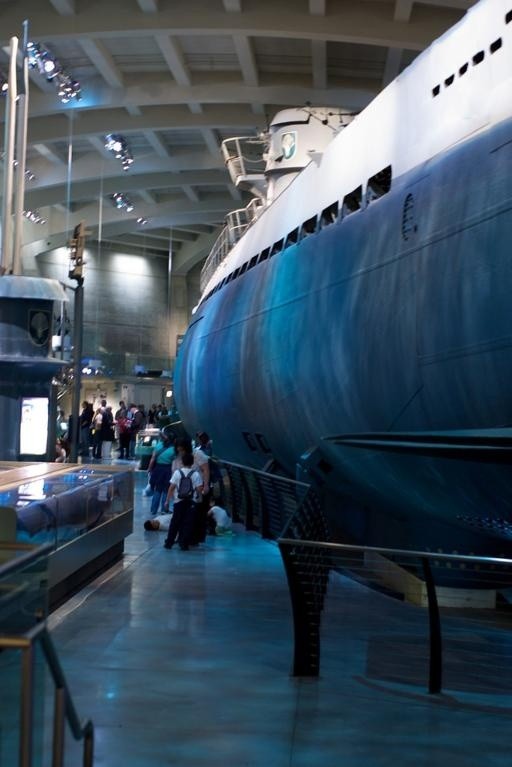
[54,397,230,552]
[281,133,295,159]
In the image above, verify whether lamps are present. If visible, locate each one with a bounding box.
[1,81,22,105]
[0,134,150,230]
[25,42,84,107]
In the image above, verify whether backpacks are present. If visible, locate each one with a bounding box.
[177,469,196,499]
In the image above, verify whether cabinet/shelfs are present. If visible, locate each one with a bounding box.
[0,460,137,635]
